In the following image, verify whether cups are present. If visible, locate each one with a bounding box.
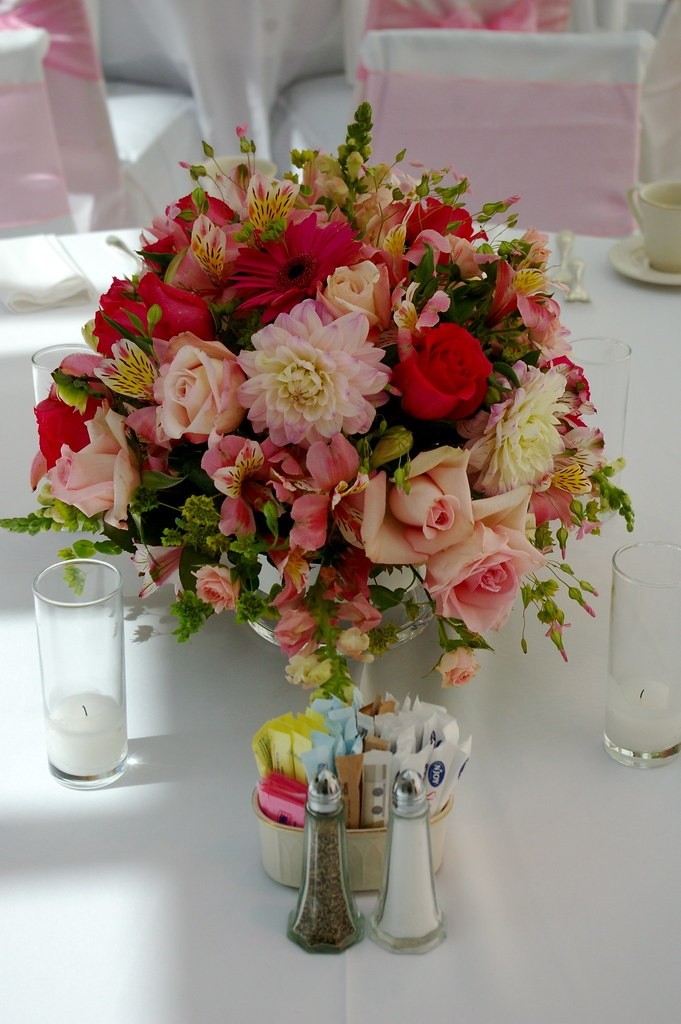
[567,337,632,523]
[603,541,681,769]
[627,181,681,272]
[30,559,128,789]
[31,343,106,408]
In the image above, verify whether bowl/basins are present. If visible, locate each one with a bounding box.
[252,785,453,889]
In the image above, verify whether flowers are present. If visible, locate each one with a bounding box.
[1,101,636,706]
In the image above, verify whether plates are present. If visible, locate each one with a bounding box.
[607,236,681,286]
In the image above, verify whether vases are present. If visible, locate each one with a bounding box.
[243,552,437,660]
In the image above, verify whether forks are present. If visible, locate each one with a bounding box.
[550,230,591,304]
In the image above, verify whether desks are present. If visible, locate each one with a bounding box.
[0,232,681,1022]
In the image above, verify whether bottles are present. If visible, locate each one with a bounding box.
[367,769,449,954]
[286,763,364,955]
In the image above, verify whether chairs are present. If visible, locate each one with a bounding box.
[0,0,681,237]
